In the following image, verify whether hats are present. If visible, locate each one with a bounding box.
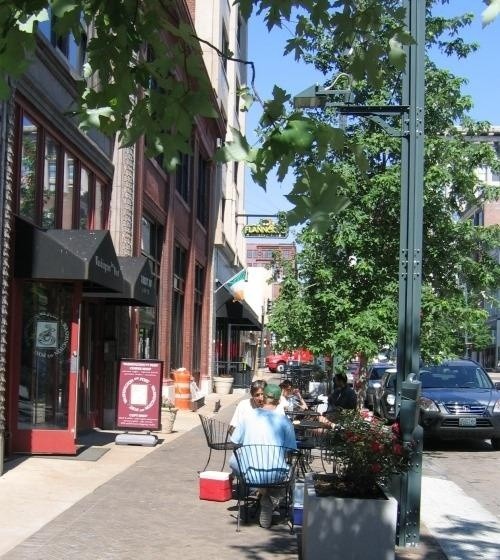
[263,384,281,400]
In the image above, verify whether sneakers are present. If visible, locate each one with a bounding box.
[259,495,274,528]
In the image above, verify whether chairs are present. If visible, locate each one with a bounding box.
[196,374,348,532]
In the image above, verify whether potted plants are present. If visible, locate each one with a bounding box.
[152,395,180,434]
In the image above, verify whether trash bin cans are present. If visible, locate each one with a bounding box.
[294,369,312,393]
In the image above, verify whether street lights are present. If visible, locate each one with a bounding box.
[294,69,431,548]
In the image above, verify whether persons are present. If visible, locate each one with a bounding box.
[229,373,359,438]
[228,384,298,529]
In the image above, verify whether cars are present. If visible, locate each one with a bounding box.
[264,345,500,453]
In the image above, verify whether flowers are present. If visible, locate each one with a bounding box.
[320,408,422,498]
